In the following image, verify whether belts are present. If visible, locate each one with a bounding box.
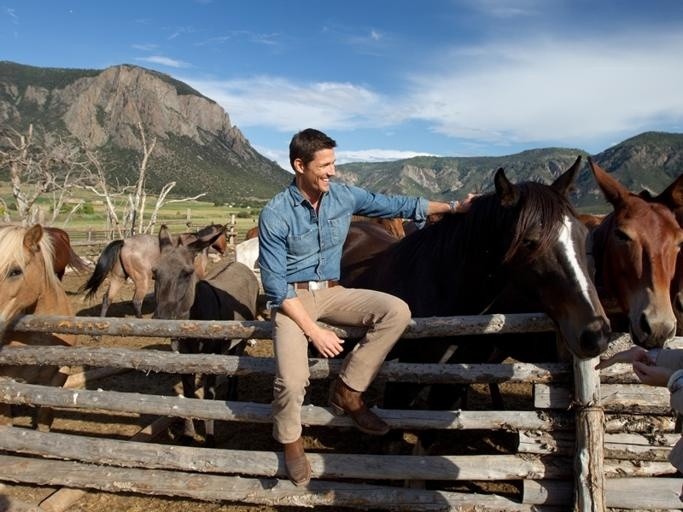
[291,281,340,288]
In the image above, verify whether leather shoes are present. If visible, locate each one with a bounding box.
[284,436,311,486]
[329,376,390,435]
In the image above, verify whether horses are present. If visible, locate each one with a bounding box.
[0,224,77,433]
[78,221,230,319]
[348,209,405,240]
[337,154,613,491]
[151,224,261,447]
[508,156,683,365]
[43,226,90,281]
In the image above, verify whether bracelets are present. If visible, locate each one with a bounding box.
[449,199,464,214]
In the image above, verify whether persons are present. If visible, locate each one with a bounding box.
[258,128,483,486]
